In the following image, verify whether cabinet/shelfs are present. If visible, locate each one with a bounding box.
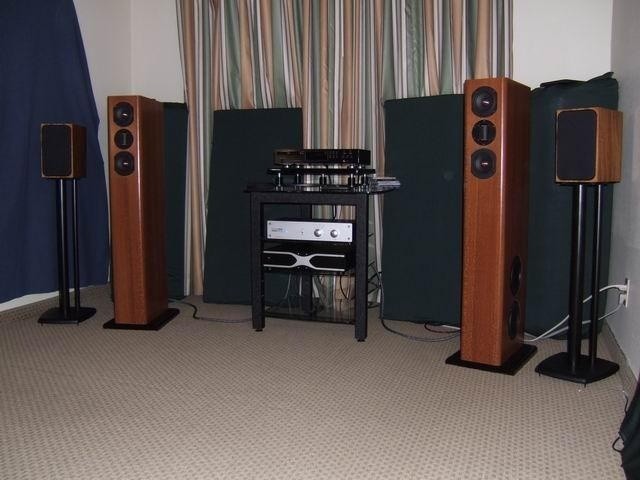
[242,184,396,342]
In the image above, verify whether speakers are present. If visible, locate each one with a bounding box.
[459,78,531,367]
[39,121,87,180]
[107,95,168,326]
[555,107,623,185]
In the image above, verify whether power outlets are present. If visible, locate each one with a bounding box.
[623,279,630,309]
